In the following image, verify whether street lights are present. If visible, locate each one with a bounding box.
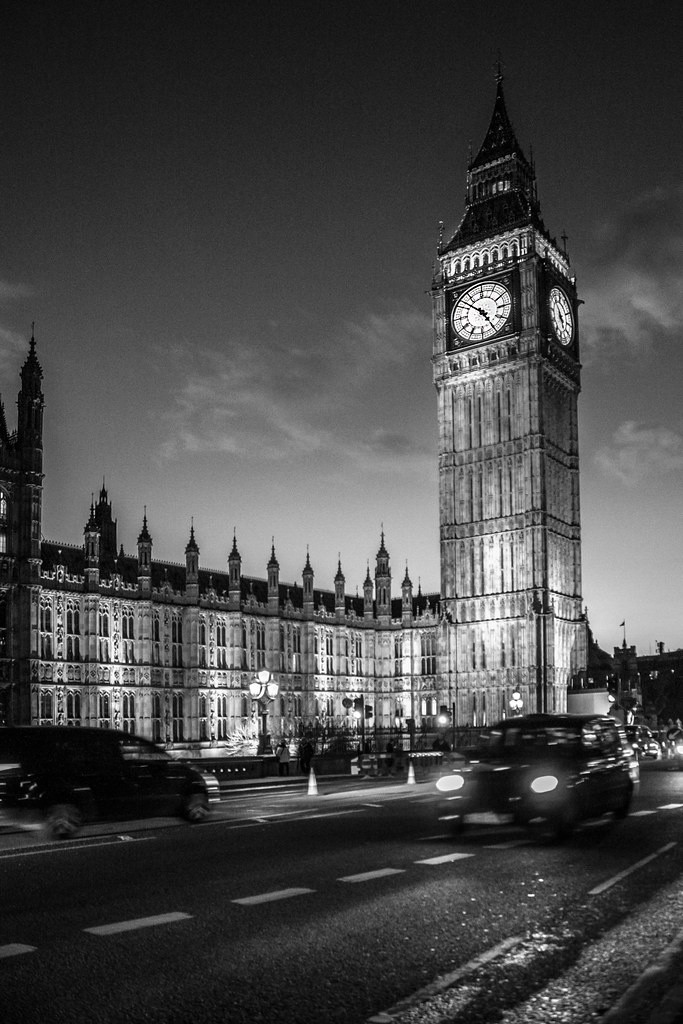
[248,670,278,756]
[508,685,524,716]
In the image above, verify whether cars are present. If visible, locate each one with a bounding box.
[0,725,221,840]
[432,712,643,825]
[624,722,663,761]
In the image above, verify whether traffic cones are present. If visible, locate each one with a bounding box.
[407,760,417,784]
[307,766,319,795]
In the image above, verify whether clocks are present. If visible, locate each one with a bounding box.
[549,285,576,347]
[451,280,512,343]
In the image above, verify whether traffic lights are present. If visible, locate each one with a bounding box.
[364,704,376,720]
[353,697,364,711]
